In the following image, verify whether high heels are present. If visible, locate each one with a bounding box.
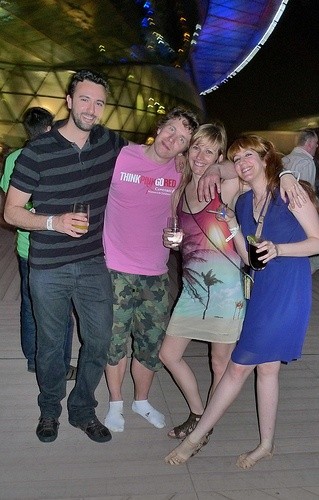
[237,445,274,470]
[164,437,199,466]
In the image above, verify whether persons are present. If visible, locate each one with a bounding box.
[165,133,319,467]
[2,67,188,441]
[279,128,318,273]
[103,98,240,432]
[0,105,81,381]
[157,119,309,440]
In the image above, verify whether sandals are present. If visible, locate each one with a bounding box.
[168,412,202,439]
[182,427,213,457]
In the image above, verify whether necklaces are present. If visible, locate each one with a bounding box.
[252,185,269,208]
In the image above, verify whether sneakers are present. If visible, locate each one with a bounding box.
[77,415,112,442]
[36,418,58,442]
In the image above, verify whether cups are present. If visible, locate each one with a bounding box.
[248,235,269,271]
[166,215,184,247]
[71,202,90,233]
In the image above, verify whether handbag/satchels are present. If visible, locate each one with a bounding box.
[244,275,254,300]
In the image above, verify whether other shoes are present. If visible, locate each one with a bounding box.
[65,366,77,380]
[27,368,36,374]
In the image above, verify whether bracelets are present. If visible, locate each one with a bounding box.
[46,214,53,231]
